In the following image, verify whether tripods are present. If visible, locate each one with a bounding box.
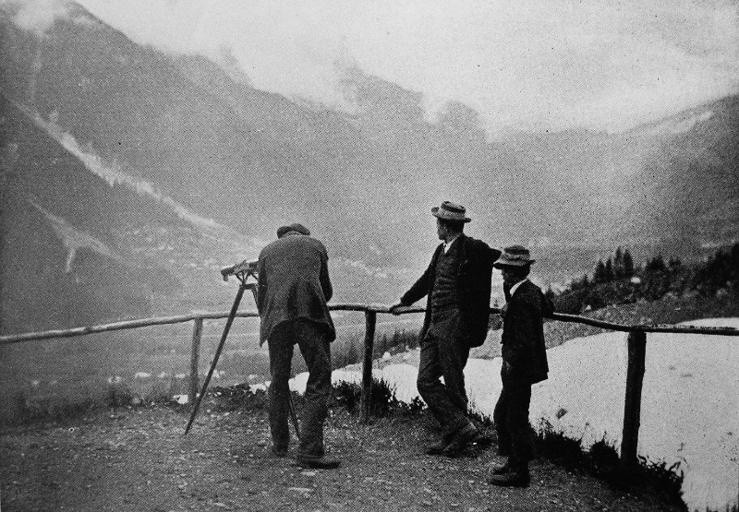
[183,282,300,442]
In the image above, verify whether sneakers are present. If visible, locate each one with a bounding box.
[443,418,477,452]
[298,457,340,469]
[271,445,286,456]
[426,438,450,452]
[489,466,531,487]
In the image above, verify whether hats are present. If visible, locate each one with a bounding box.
[494,245,536,269]
[277,222,310,236]
[431,200,471,222]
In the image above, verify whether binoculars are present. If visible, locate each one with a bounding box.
[220,260,258,282]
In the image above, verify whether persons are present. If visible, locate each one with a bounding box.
[488,245,557,488]
[253,223,341,469]
[386,202,493,457]
